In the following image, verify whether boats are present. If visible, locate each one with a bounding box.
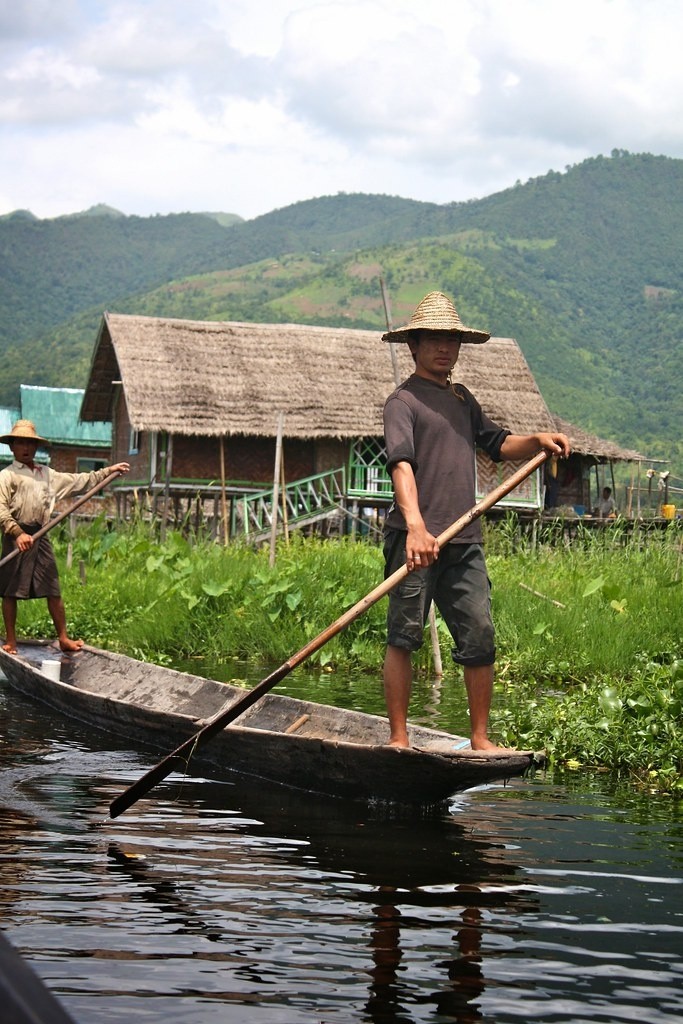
[0,633,551,806]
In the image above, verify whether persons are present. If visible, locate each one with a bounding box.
[0,420,130,653]
[382,291,570,752]
[593,486,618,518]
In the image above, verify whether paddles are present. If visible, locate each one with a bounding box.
[0,465,126,568]
[105,440,566,824]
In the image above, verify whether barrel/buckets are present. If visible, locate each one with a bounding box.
[573,504,585,516]
[662,505,676,518]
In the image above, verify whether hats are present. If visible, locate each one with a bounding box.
[0,420,52,446]
[381,291,490,344]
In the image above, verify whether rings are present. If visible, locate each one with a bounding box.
[413,556,421,559]
